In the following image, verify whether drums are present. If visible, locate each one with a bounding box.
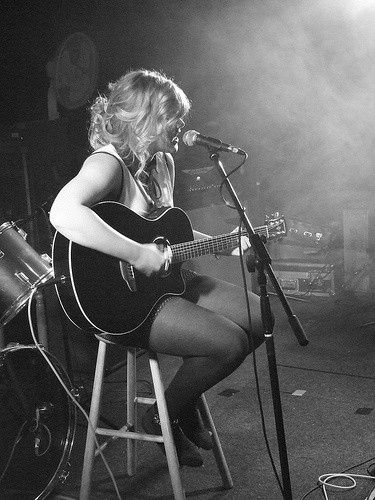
[0,221,54,329]
[0,342,78,500]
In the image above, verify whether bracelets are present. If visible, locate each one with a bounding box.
[214,254,221,260]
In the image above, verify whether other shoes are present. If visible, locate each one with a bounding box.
[175,408,213,452]
[141,407,204,467]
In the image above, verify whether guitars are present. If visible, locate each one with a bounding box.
[50,199,288,346]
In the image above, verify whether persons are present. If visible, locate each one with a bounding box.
[49,70,276,467]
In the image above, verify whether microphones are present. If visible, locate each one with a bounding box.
[182,130,246,154]
[34,424,44,451]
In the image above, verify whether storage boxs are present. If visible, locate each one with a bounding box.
[251,258,342,298]
[342,208,375,293]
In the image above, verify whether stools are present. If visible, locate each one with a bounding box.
[79,335,234,500]
